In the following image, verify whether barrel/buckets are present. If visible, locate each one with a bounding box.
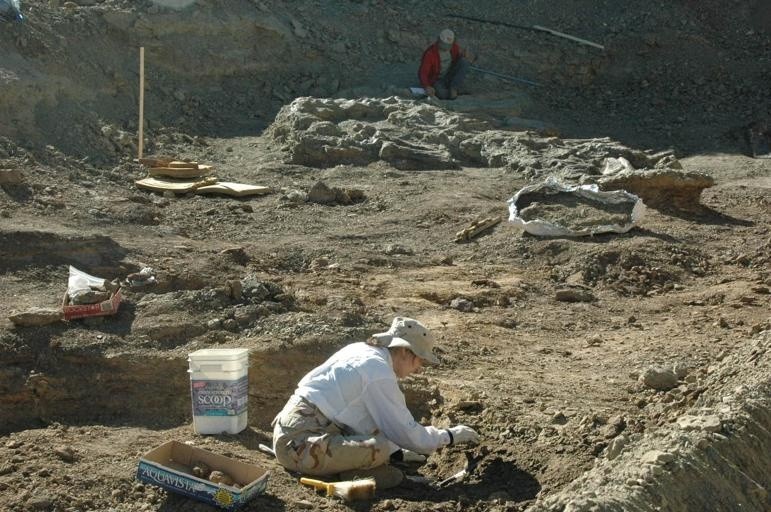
[186,348,249,437]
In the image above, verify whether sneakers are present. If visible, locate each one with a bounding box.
[340,464,404,490]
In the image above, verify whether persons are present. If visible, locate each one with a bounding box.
[418,28,472,100]
[269,315,481,489]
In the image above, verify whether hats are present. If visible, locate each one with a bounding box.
[440,29,455,45]
[372,316,442,365]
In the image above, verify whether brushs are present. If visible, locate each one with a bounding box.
[300,476,377,503]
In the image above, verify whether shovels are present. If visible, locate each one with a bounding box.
[238,429,274,455]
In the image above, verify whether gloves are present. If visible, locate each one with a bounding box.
[402,448,427,462]
[447,423,482,448]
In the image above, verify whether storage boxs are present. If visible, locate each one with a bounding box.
[61,285,122,320]
[138,440,269,512]
